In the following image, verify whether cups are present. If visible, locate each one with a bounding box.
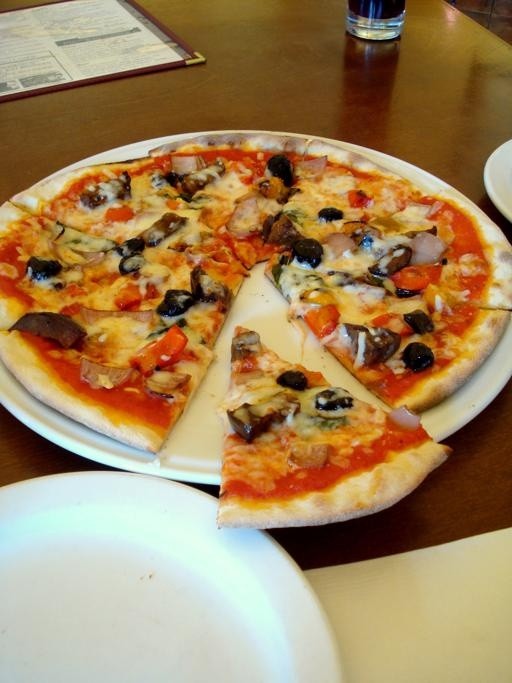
[344,36,400,111]
[345,0,408,41]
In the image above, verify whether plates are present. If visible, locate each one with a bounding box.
[481,135,512,224]
[0,470,347,682]
